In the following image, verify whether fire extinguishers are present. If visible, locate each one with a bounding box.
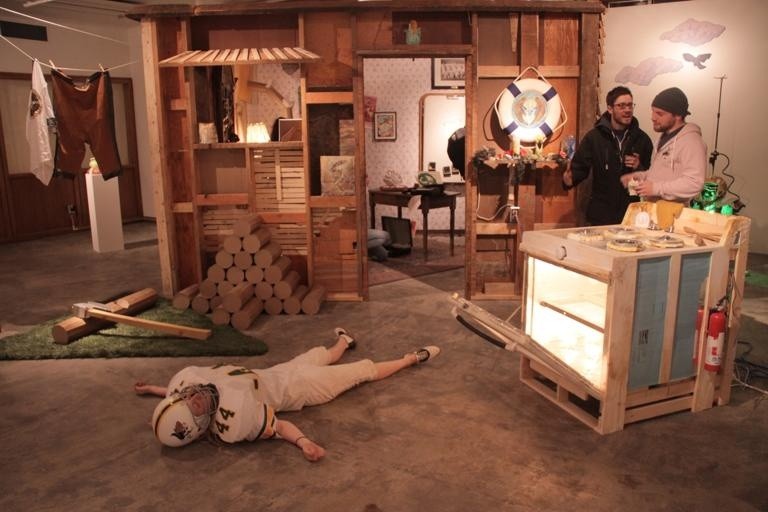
[692,295,729,371]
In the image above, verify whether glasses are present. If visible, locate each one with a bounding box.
[611,102,636,110]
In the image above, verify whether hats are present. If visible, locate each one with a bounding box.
[651,87,691,115]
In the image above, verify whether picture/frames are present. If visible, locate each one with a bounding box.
[374,111,398,141]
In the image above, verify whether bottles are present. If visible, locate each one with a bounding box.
[88,158,101,173]
[567,135,576,159]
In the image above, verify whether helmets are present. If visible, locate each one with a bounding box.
[150,384,218,448]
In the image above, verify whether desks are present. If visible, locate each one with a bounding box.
[367,185,461,264]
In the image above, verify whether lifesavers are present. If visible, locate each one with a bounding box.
[498,78,561,143]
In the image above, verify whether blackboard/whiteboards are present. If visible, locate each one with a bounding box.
[419,94,467,182]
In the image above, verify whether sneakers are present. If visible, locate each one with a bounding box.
[405,345,442,363]
[332,326,357,348]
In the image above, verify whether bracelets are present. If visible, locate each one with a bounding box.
[296,436,308,445]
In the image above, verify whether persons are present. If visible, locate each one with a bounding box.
[134,327,441,462]
[562,86,653,226]
[620,88,707,208]
